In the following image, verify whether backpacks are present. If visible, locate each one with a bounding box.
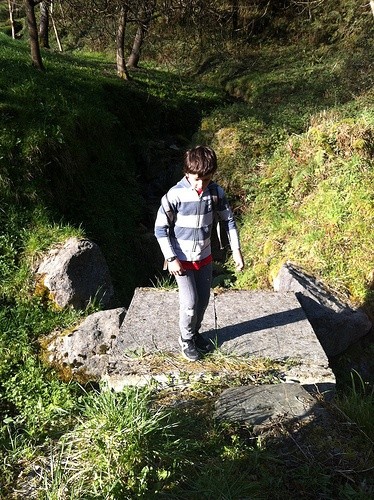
[160,181,228,264]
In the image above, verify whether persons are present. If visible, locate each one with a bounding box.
[154,144,244,362]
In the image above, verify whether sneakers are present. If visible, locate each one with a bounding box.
[193,333,209,352]
[179,336,199,361]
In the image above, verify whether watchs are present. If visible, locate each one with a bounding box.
[166,256,177,262]
